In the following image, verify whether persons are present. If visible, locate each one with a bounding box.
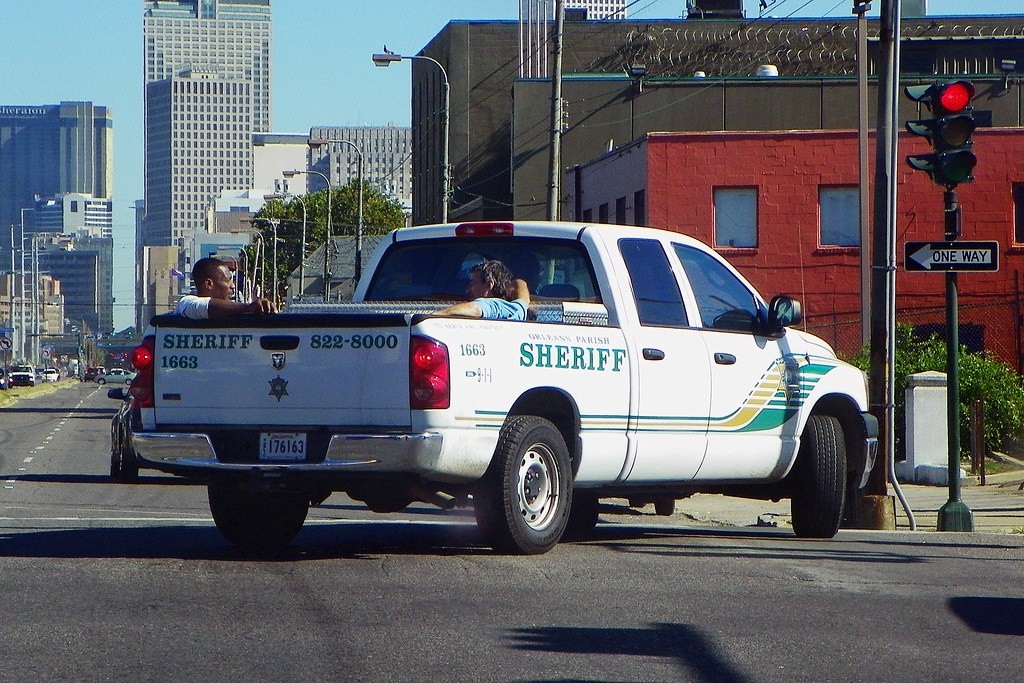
[507,250,542,292]
[173,258,280,320]
[429,258,530,321]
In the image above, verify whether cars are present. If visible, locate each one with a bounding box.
[0,362,61,390]
[93,369,136,385]
[83,366,107,381]
[104,370,136,483]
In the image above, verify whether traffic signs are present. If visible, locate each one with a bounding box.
[903,241,1002,276]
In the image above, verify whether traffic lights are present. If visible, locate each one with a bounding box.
[901,83,941,179]
[929,80,979,187]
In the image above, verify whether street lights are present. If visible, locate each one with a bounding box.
[285,169,332,293]
[263,191,307,301]
[209,254,238,305]
[371,52,452,231]
[239,217,277,308]
[229,228,266,303]
[214,244,249,305]
[304,138,366,288]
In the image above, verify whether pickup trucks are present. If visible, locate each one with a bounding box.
[120,218,882,556]
